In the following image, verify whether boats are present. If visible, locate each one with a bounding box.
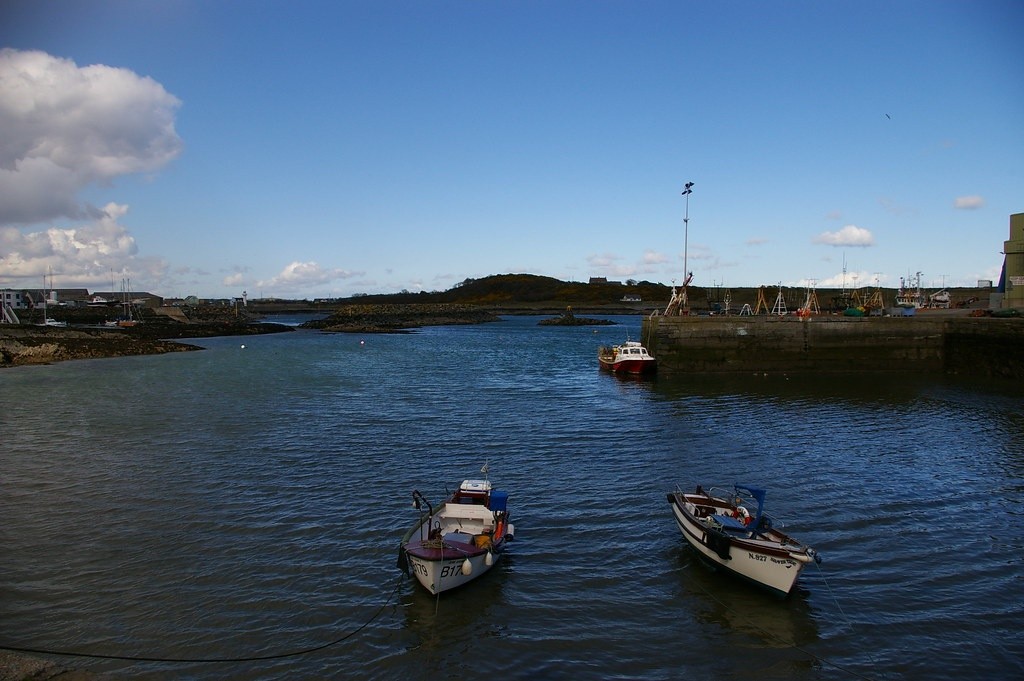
[664,270,953,319]
[665,480,821,599]
[395,454,515,596]
[598,340,655,375]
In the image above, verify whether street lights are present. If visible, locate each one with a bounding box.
[682,179,695,285]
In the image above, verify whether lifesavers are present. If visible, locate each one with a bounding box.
[733,506,751,527]
